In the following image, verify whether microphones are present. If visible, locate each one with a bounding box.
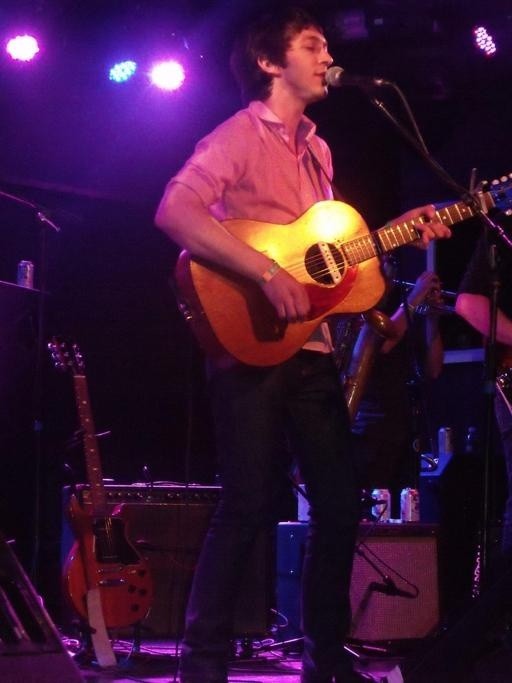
[324,65,384,89]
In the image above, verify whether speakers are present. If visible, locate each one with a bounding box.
[60,483,274,639]
[274,521,442,645]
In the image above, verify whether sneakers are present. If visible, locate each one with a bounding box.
[301,669,373,683]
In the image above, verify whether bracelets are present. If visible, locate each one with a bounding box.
[255,263,281,287]
[400,301,418,313]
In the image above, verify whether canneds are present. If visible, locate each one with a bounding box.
[17,260,34,289]
[372,488,390,522]
[401,488,420,523]
[438,427,450,454]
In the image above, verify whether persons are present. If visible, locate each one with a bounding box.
[153,6,453,683]
[366,267,448,510]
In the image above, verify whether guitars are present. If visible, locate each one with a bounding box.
[47,341,156,628]
[172,170,512,368]
